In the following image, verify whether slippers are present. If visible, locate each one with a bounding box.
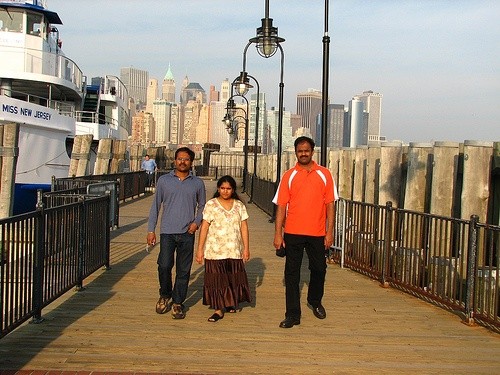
[207,312,224,322]
[227,306,236,313]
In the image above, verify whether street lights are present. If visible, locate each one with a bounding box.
[249,0,330,167]
[231,40,284,222]
[221,94,250,194]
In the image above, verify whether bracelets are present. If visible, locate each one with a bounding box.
[148,231,153,233]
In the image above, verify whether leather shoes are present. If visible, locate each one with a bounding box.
[279,316,300,328]
[307,301,326,319]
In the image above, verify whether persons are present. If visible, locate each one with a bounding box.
[196,175,251,322]
[141,155,157,185]
[272,136,340,328]
[146,147,206,320]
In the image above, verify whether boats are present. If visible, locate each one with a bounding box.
[0,0,132,216]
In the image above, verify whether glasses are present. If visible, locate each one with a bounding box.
[177,156,191,161]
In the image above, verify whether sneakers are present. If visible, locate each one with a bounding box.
[155,296,173,314]
[170,303,184,319]
